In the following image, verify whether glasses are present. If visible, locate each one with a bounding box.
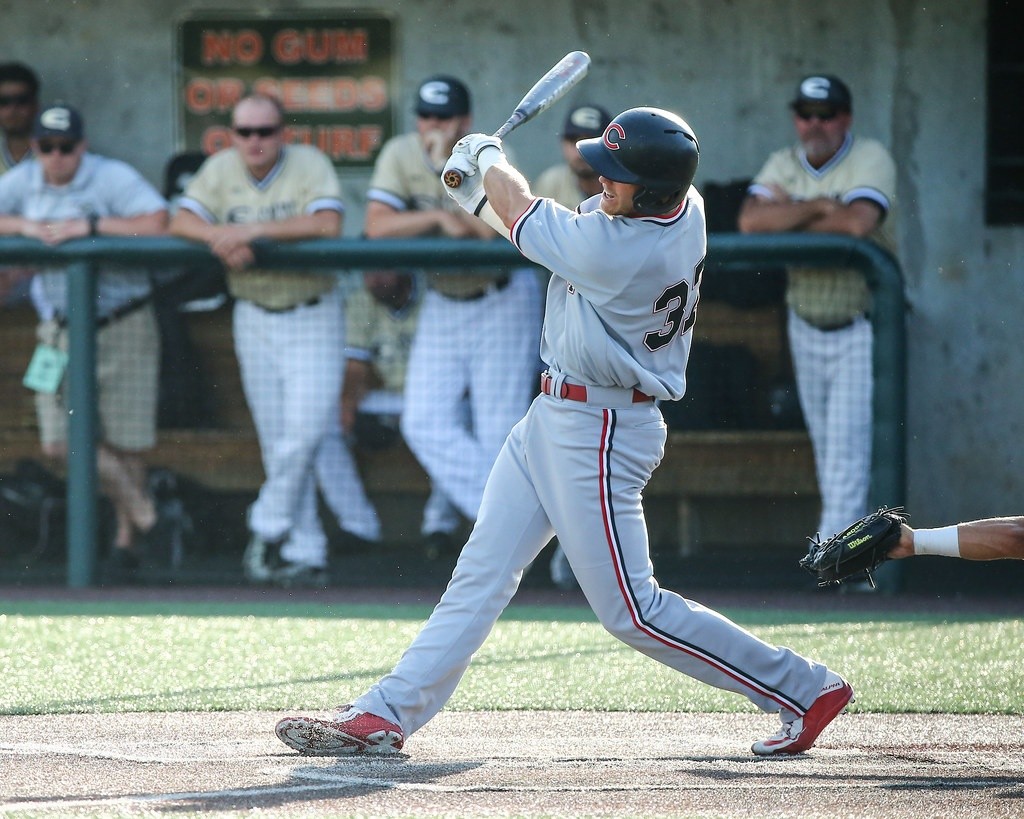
[0,94,33,107]
[230,124,280,138]
[38,140,76,154]
[795,107,839,120]
[567,135,582,143]
[419,111,453,119]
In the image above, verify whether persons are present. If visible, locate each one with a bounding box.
[315,229,469,569]
[799,506,1024,588]
[0,59,171,576]
[341,73,549,571]
[275,106,856,766]
[169,93,389,583]
[737,72,899,589]
[529,106,624,592]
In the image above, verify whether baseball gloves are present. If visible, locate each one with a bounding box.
[798,511,909,590]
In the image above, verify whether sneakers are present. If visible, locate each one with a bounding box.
[273,703,406,757]
[750,669,855,757]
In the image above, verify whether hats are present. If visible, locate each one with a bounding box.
[30,103,85,145]
[560,103,612,138]
[415,77,469,114]
[789,73,852,111]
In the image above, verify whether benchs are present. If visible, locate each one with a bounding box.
[1,303,819,558]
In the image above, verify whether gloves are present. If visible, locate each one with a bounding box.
[441,133,502,219]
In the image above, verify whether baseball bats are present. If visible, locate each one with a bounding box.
[443,50,593,189]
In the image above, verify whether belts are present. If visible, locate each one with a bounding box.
[252,297,320,314]
[803,311,871,332]
[57,297,144,331]
[439,275,509,301]
[540,373,655,403]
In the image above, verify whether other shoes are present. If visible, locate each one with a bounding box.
[104,550,150,582]
[239,538,333,588]
[145,516,182,576]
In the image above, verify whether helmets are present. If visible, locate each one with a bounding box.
[575,107,701,216]
